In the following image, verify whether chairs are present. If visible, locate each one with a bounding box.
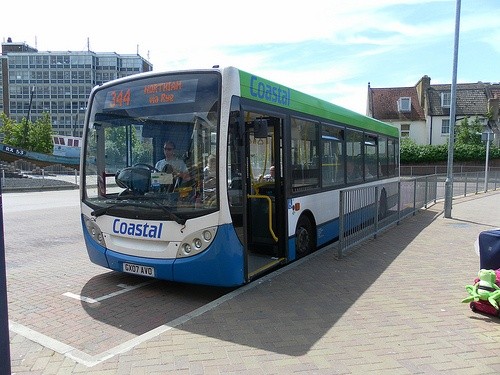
[293,153,394,187]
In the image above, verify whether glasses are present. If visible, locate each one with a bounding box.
[164,147,173,151]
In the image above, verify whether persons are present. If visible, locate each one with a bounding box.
[204,154,216,198]
[264,166,275,182]
[154,140,191,193]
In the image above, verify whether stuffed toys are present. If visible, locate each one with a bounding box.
[461,269,500,310]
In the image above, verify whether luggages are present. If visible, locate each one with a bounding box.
[470,269,500,316]
[479,230,500,270]
